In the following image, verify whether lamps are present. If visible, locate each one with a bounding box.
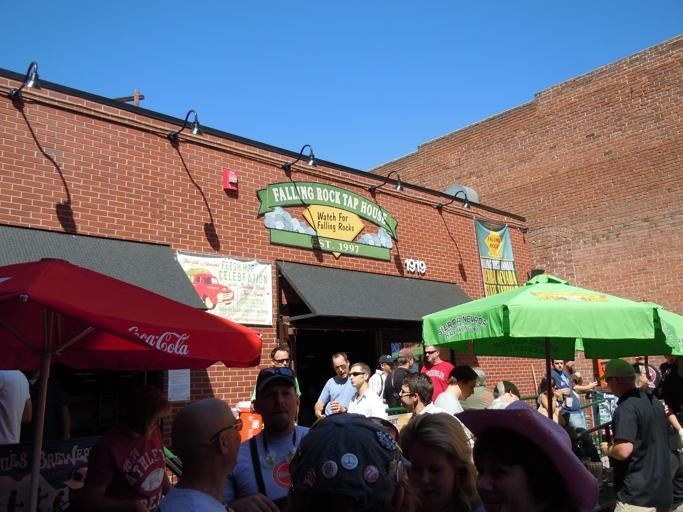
[368,170,405,194]
[436,190,470,210]
[7,62,43,102]
[281,143,317,174]
[167,109,203,144]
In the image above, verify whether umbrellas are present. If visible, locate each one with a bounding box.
[421,275,683,419]
[0,259,264,512]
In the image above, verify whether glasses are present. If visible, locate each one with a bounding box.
[348,370,366,377]
[257,367,294,387]
[271,356,290,363]
[333,364,347,371]
[425,350,436,355]
[210,418,244,442]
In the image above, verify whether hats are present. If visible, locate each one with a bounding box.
[398,349,421,363]
[378,354,398,366]
[456,400,599,511]
[287,412,412,504]
[597,359,635,381]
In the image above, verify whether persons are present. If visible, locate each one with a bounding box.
[78,367,600,512]
[1,369,70,444]
[537,353,682,511]
[251,346,301,426]
[315,344,520,463]
